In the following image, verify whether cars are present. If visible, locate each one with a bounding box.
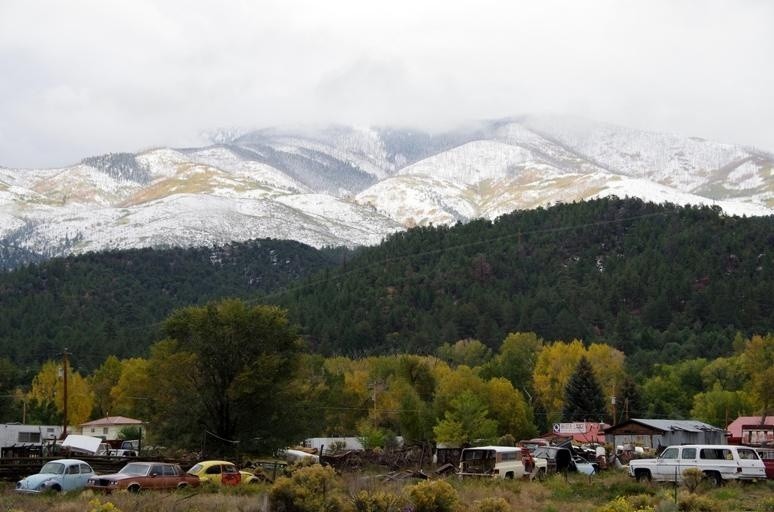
[88,462,200,495]
[188,460,261,489]
[97,440,143,461]
[16,459,97,497]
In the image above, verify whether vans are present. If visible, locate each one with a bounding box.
[628,445,774,488]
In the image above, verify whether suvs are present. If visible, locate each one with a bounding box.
[459,439,573,483]
